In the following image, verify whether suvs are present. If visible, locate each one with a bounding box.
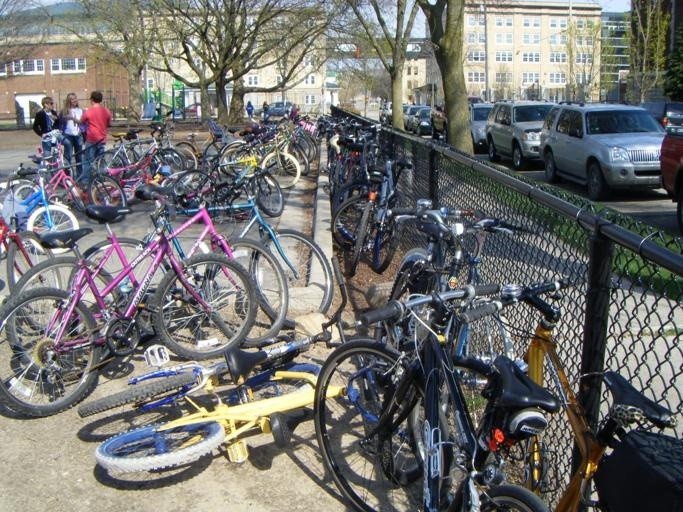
[253,102,295,119]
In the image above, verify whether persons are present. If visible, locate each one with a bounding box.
[290,104,300,120]
[430,105,449,142]
[246,101,254,120]
[407,94,415,105]
[262,101,271,125]
[49,92,87,176]
[33,97,72,167]
[64,91,113,181]
